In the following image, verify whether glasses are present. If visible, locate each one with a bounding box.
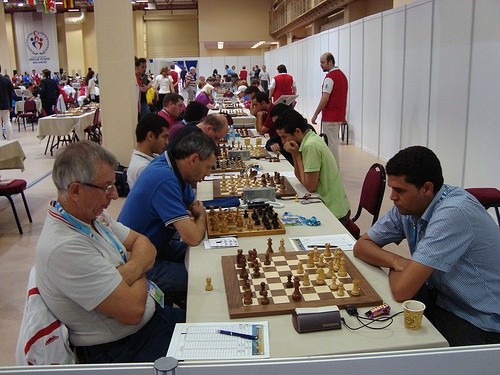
[68,180,115,193]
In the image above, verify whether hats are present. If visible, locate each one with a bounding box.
[234,86,247,94]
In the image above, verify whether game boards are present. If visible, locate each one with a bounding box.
[205,206,287,239]
[51,102,100,117]
[212,105,244,110]
[214,89,237,104]
[221,246,384,320]
[212,146,272,158]
[221,113,247,116]
[218,127,255,142]
[210,155,246,174]
[213,175,298,199]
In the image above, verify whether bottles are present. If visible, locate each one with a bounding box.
[228,125,235,144]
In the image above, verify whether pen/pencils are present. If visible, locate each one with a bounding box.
[215,329,259,340]
[308,244,338,247]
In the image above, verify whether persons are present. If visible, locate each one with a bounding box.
[311,52,348,169]
[0,56,297,191]
[117,132,219,311]
[273,111,351,228]
[35,140,186,364]
[352,146,500,348]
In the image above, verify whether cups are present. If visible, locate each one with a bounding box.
[244,138,250,145]
[402,300,426,330]
[256,137,262,145]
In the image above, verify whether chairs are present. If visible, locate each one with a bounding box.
[84,108,100,145]
[0,179,32,235]
[341,163,387,240]
[17,100,36,132]
[321,118,348,145]
[463,188,500,231]
[16,264,79,365]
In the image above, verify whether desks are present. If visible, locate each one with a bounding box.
[0,82,449,360]
